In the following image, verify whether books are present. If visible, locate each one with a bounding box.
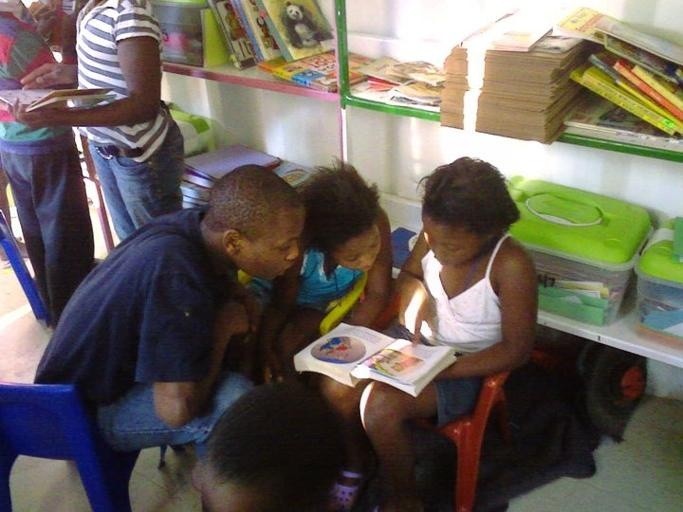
[257,49,376,93]
[255,0,337,63]
[0,88,117,113]
[180,142,281,213]
[200,1,338,71]
[293,322,456,398]
[552,7,683,151]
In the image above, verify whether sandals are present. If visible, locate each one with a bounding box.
[327,472,365,511]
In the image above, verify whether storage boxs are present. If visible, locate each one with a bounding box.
[150,1,209,67]
[499,174,654,325]
[633,217,683,340]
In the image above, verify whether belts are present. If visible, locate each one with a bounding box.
[94,145,142,159]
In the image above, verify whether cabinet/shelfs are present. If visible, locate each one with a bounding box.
[82,0,345,264]
[340,0,683,368]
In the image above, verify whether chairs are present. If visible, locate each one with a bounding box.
[419,350,549,511]
[0,381,110,512]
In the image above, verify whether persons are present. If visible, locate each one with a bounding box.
[250,162,393,395]
[318,156,538,511]
[34,165,307,491]
[196,379,346,511]
[6,0,185,241]
[0,1,96,333]
